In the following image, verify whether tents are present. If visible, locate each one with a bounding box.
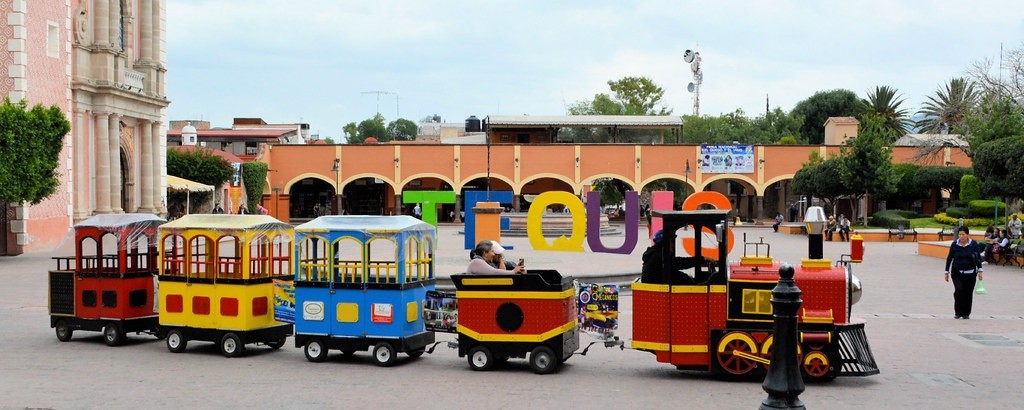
[166,175,216,214]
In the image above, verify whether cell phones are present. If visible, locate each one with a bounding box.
[519,259,524,272]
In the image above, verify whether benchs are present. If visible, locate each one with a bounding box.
[887,226,918,242]
[937,227,959,241]
[989,240,1024,269]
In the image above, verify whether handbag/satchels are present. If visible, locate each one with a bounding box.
[974,279,988,296]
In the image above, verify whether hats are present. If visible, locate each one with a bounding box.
[1013,212,1017,215]
[652,229,677,242]
[490,241,505,254]
[829,216,833,219]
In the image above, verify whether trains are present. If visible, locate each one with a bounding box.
[48,209,880,384]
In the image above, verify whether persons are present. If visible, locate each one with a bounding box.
[238,204,248,214]
[640,204,651,224]
[212,204,223,214]
[944,224,984,320]
[413,203,422,220]
[168,203,185,216]
[824,214,850,242]
[614,207,625,217]
[642,229,716,285]
[984,214,1023,264]
[773,212,784,232]
[732,215,746,225]
[466,240,527,274]
[789,204,798,222]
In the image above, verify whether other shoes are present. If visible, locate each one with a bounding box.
[954,314,960,319]
[982,261,989,265]
[981,253,985,256]
[963,315,969,319]
